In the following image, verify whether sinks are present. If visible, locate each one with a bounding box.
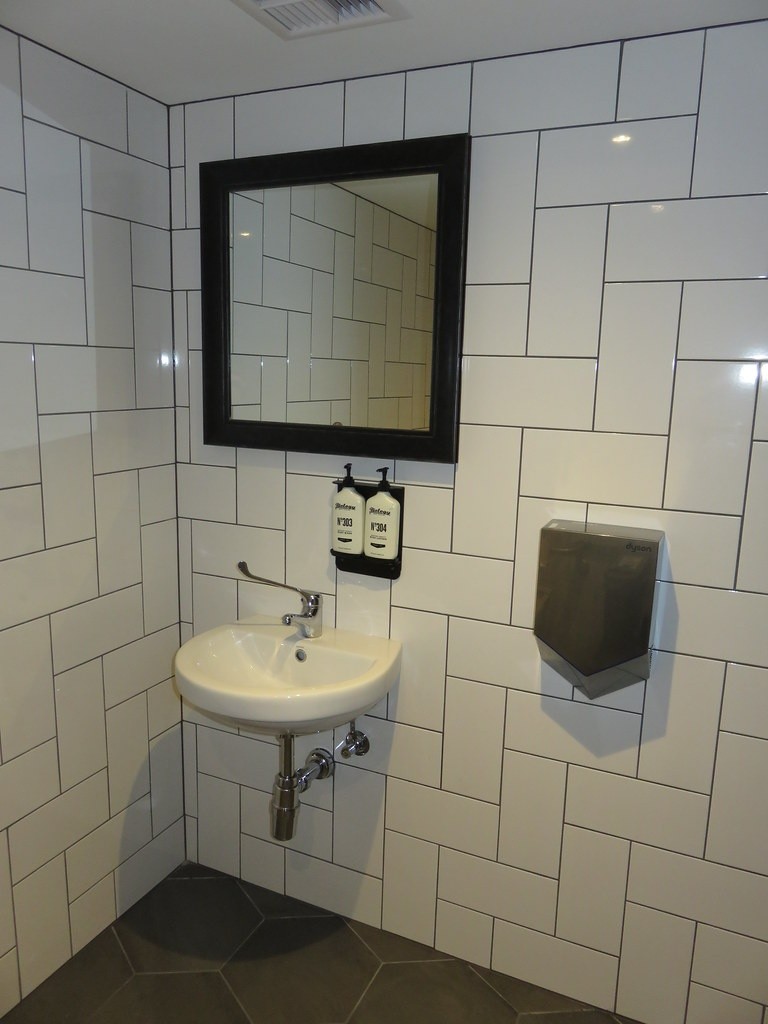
[175,614,402,735]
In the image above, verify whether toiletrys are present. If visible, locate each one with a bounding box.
[364,467,399,559]
[333,463,365,555]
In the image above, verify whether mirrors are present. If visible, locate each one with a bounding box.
[197,131,473,465]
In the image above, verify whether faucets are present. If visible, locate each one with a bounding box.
[237,561,322,638]
